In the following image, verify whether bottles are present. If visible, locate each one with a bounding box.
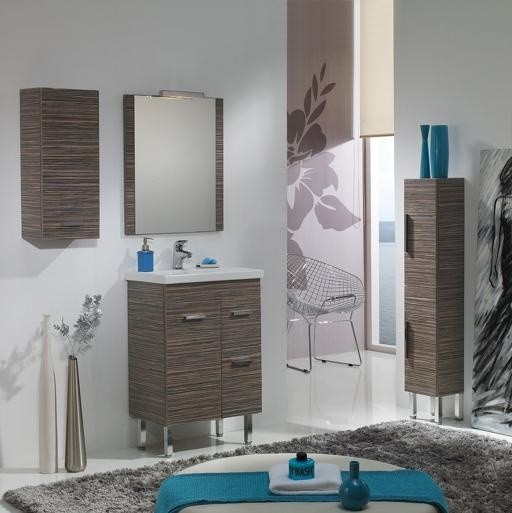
[420,124,431,177]
[338,459,370,512]
[288,450,315,480]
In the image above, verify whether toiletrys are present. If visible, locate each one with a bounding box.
[137,237,154,272]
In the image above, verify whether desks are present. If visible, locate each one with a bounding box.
[177,452,437,512]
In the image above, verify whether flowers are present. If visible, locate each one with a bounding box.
[51,294,102,356]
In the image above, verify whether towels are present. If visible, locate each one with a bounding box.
[155,468,449,512]
[268,462,342,496]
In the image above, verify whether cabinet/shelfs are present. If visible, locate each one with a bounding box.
[128,278,262,457]
[19,87,99,241]
[403,178,465,425]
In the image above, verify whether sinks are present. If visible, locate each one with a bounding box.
[160,268,223,273]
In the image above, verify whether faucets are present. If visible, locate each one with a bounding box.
[173,240,192,269]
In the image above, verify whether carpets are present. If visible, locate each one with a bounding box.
[3,418,512,512]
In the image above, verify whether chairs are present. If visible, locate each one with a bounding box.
[286,253,366,373]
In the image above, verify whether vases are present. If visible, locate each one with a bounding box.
[65,355,87,472]
[419,124,430,178]
[38,314,58,474]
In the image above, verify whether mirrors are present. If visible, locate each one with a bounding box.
[122,94,224,235]
